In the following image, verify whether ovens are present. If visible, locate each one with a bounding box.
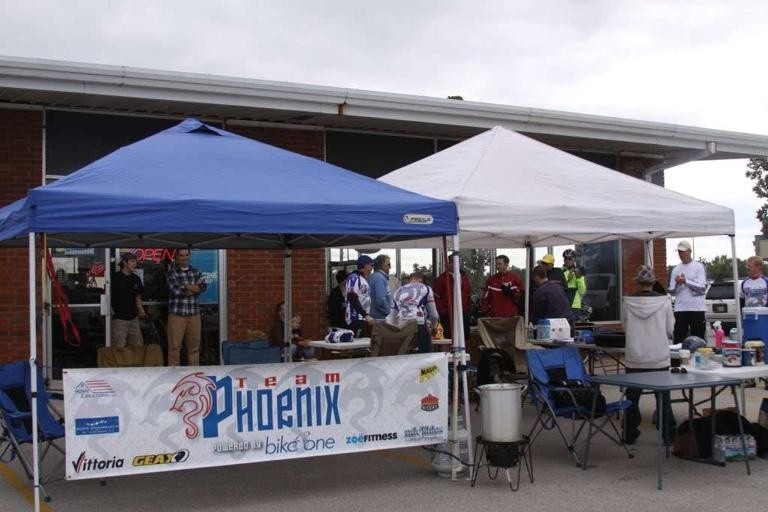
[705,298,741,340]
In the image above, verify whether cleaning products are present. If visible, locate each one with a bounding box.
[713,321,724,348]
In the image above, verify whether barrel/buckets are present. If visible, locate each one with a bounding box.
[741,307,768,363]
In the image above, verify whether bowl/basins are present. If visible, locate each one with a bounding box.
[592,331,625,347]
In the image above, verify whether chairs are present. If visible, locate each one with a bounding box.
[474,316,545,412]
[0,359,107,502]
[367,319,418,358]
[222,340,281,365]
[522,346,634,471]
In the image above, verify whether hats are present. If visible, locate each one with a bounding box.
[537,255,554,265]
[357,256,376,264]
[676,241,691,251]
[118,253,137,266]
[633,264,656,283]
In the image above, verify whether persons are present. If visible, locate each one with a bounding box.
[434,252,473,338]
[111,252,145,348]
[621,264,677,445]
[481,249,589,337]
[165,249,208,366]
[739,256,768,314]
[668,242,708,344]
[272,301,314,362]
[327,255,440,353]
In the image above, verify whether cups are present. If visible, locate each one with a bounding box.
[670,349,690,366]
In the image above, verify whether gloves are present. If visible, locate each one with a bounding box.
[501,283,513,297]
[574,268,582,277]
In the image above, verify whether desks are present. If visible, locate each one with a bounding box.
[307,336,452,360]
[527,338,768,490]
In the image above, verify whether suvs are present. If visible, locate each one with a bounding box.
[700,273,747,328]
[577,264,616,324]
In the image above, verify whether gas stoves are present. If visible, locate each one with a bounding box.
[470,436,533,491]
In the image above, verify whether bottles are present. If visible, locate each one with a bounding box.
[695,338,766,370]
[712,433,757,463]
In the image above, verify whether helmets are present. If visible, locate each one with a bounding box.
[563,249,575,257]
[682,336,706,353]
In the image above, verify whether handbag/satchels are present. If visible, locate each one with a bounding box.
[325,327,355,342]
[548,378,606,419]
